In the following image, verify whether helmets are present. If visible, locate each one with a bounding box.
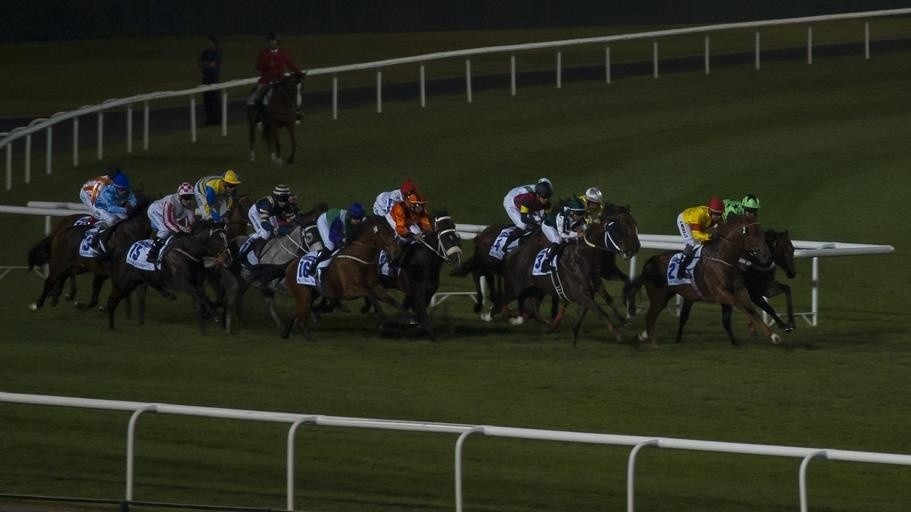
[536,178,602,210]
[177,182,194,201]
[109,167,129,189]
[348,203,365,223]
[708,197,725,213]
[272,184,290,200]
[742,195,760,210]
[223,170,241,190]
[401,180,426,216]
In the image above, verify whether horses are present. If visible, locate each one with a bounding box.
[27,213,157,311]
[248,70,305,164]
[107,216,235,333]
[360,207,463,325]
[674,229,797,345]
[222,203,330,336]
[448,194,642,346]
[231,211,419,341]
[622,211,784,345]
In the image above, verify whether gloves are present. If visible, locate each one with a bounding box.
[711,232,719,240]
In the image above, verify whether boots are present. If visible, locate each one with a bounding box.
[240,237,265,263]
[309,247,331,275]
[146,237,162,263]
[678,244,695,278]
[89,224,105,255]
[502,227,524,253]
[541,242,560,272]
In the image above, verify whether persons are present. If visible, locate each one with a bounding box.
[88,173,140,257]
[146,181,198,264]
[371,179,419,217]
[539,195,590,271]
[676,196,727,279]
[307,203,368,277]
[501,176,554,255]
[238,181,302,269]
[719,193,761,226]
[577,186,605,225]
[78,165,121,227]
[384,191,435,270]
[198,35,224,128]
[250,30,305,124]
[194,169,242,225]
